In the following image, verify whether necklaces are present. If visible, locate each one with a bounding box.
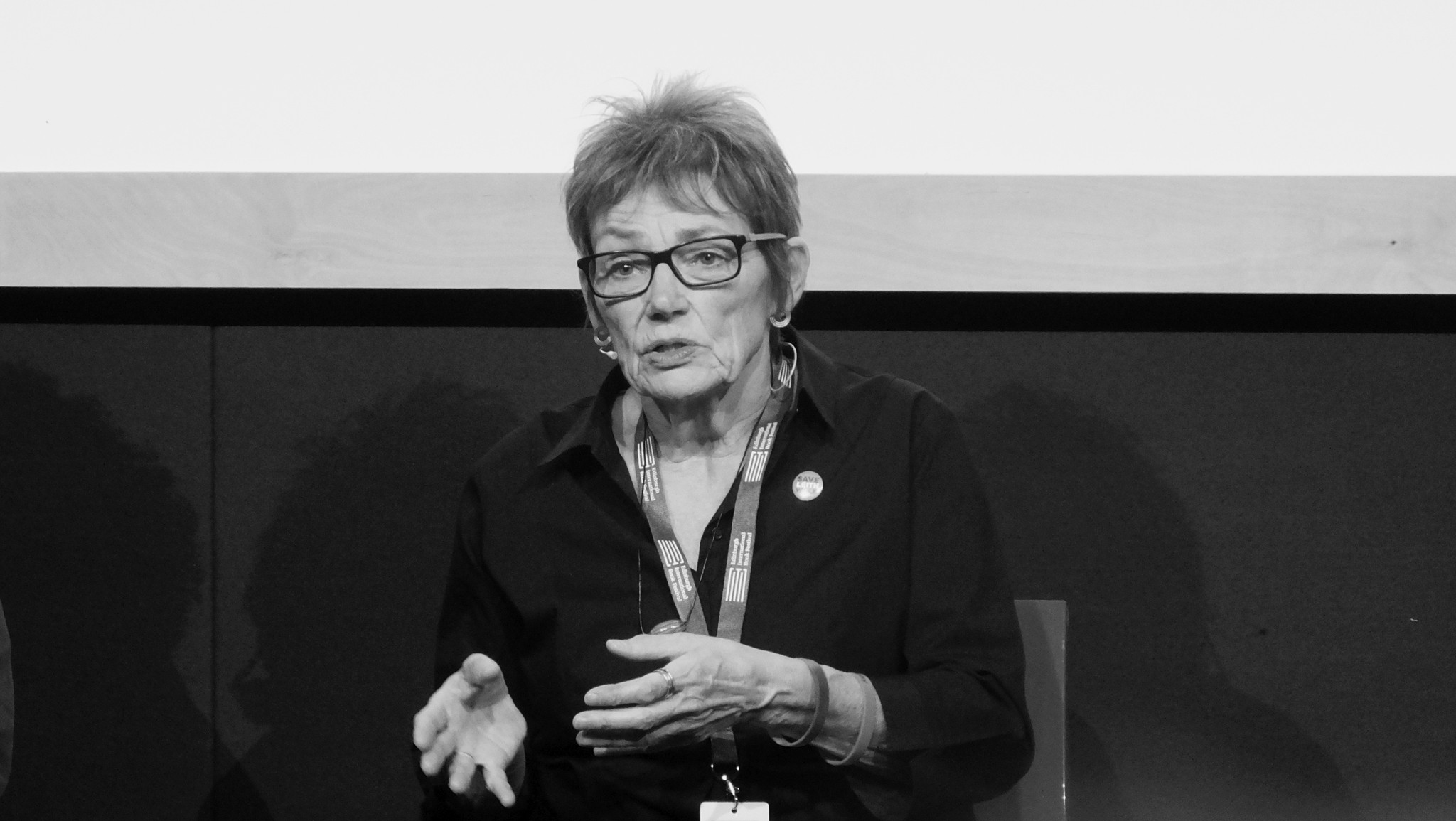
[637,393,767,634]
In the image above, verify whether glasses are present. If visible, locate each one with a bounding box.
[577,233,787,298]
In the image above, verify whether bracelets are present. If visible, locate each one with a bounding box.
[816,671,876,767]
[765,658,828,749]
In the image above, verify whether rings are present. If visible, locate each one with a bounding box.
[652,667,674,702]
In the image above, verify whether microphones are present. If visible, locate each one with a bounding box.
[599,346,618,360]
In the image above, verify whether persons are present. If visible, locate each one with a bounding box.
[410,73,1035,821]
